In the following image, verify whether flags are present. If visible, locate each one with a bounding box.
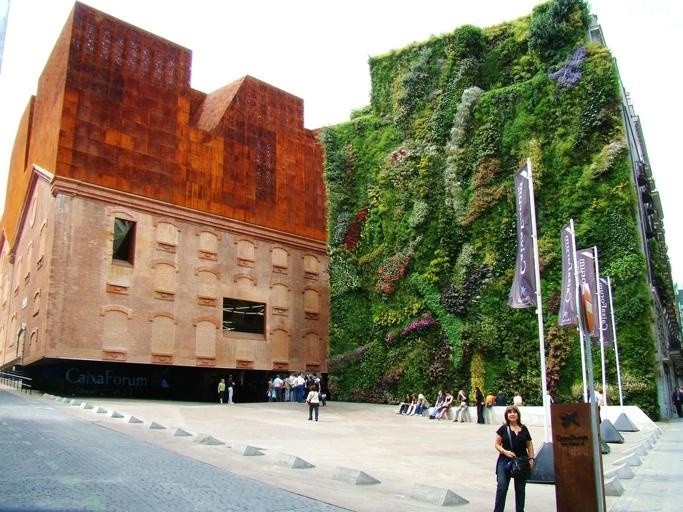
[556,221,580,328]
[505,162,539,310]
[595,276,614,348]
[575,247,599,339]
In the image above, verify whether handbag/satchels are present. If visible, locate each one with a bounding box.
[508,456,531,481]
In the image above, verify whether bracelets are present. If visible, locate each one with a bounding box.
[528,456,535,461]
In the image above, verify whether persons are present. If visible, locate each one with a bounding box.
[672,385,682,418]
[589,387,603,424]
[304,385,320,422]
[216,378,225,404]
[395,387,506,424]
[321,390,327,406]
[513,390,523,406]
[492,406,536,512]
[265,370,322,403]
[225,374,235,404]
